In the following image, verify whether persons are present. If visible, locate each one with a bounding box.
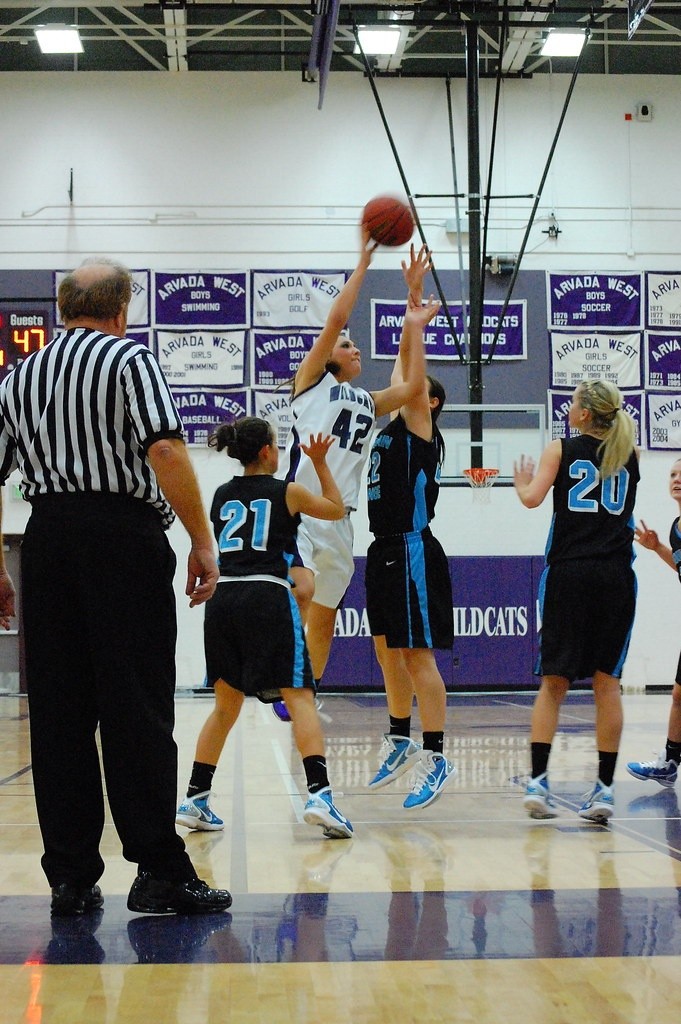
[272,225,441,722]
[174,417,354,839]
[625,457,681,788]
[513,378,641,820]
[0,257,234,916]
[366,243,455,809]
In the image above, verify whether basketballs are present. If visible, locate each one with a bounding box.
[362,198,414,246]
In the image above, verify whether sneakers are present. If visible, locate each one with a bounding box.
[298,839,354,887]
[50,908,106,940]
[366,733,423,792]
[399,824,446,880]
[51,885,105,916]
[522,779,558,820]
[626,788,679,822]
[578,776,618,823]
[583,835,618,861]
[126,872,232,913]
[367,827,416,884]
[183,831,224,868]
[302,787,354,839]
[521,828,557,872]
[625,748,678,788]
[127,910,234,963]
[400,749,458,810]
[176,797,225,831]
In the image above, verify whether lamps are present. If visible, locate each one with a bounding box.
[354,25,401,55]
[33,24,87,54]
[539,28,593,57]
